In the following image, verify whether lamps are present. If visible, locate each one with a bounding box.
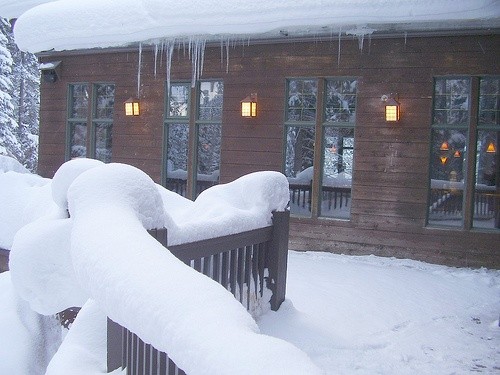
[384,92,401,127]
[239,96,257,119]
[122,97,141,118]
